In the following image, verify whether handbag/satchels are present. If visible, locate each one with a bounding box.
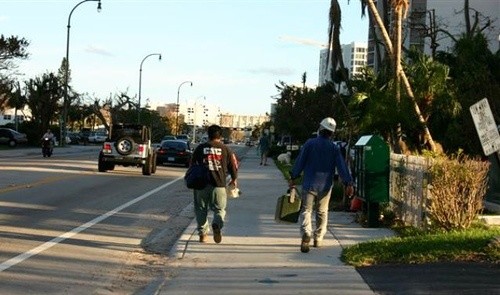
[184,164,208,190]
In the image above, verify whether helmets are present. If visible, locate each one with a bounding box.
[320,117,336,133]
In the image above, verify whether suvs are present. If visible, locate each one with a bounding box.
[98,122,168,173]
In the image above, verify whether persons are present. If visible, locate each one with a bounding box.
[41,128,54,155]
[190,125,238,243]
[288,117,353,253]
[257,133,270,166]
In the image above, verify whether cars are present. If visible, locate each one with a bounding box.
[0,128,34,146]
[64,133,84,143]
[159,134,209,147]
[156,139,192,165]
[88,130,111,143]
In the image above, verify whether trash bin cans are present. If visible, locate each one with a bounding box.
[356,134,390,203]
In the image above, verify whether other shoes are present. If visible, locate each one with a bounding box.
[200,234,208,242]
[314,241,321,247]
[301,233,310,253]
[212,222,221,243]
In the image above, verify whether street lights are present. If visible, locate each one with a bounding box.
[175,81,194,136]
[59,0,101,146]
[136,53,162,124]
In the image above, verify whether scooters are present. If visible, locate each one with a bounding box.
[42,137,55,158]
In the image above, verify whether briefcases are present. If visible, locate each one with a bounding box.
[275,186,302,224]
[226,184,240,199]
